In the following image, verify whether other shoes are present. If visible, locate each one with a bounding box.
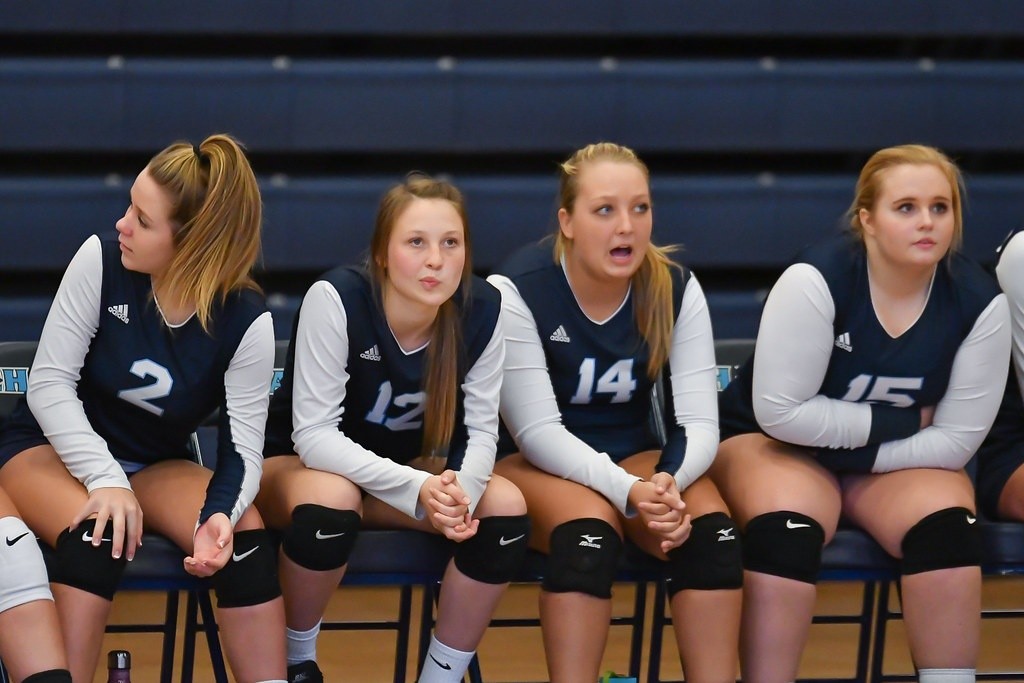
[288,659,323,683]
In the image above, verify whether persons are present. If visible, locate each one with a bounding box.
[248,170,532,683]
[708,141,1024,683]
[485,139,745,683]
[0,488,72,683]
[0,134,293,683]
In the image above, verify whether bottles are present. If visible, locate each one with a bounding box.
[106,649,131,683]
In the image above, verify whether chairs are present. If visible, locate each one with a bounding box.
[0,337,1024,683]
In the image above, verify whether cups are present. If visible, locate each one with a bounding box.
[601,671,637,683]
